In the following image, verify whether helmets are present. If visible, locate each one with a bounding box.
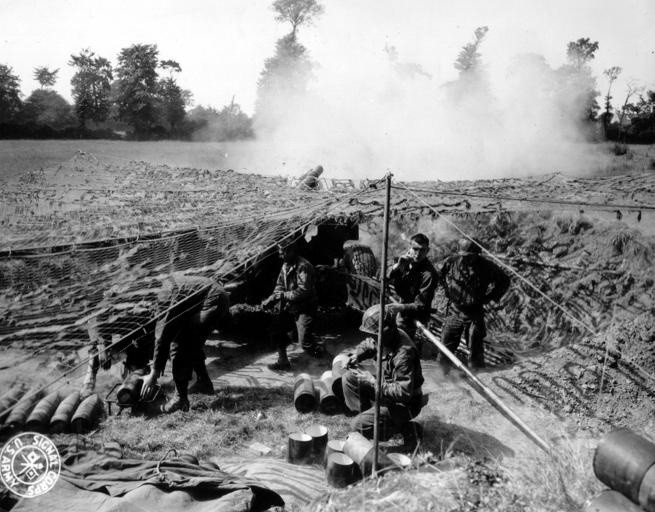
[457,236,483,256]
[359,304,399,338]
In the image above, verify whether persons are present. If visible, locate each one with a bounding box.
[136,274,231,415]
[384,233,438,341]
[261,237,329,368]
[437,236,513,377]
[80,297,152,394]
[340,303,425,450]
[338,238,376,280]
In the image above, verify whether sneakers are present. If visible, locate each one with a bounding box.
[267,360,291,371]
[187,383,214,395]
[151,402,190,414]
